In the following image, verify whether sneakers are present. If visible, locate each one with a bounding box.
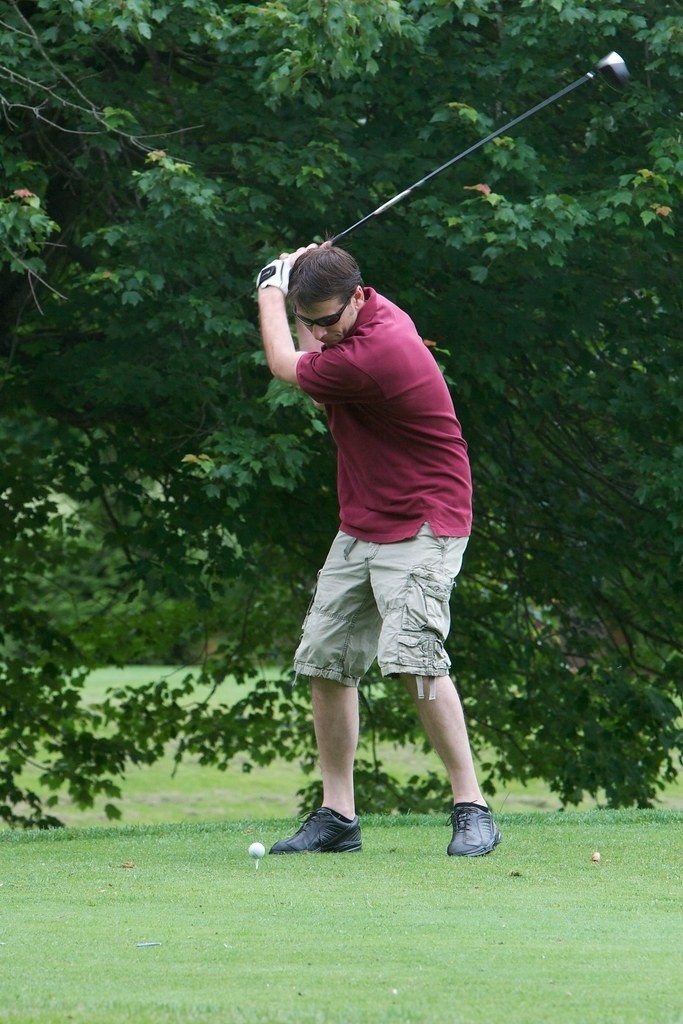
[446,805,502,856]
[269,807,363,855]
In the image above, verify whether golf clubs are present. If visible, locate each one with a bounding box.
[323,49,631,245]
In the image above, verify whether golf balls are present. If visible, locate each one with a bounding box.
[248,842,265,858]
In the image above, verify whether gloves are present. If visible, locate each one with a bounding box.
[256,256,293,298]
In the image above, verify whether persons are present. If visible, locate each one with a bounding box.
[257,239,503,856]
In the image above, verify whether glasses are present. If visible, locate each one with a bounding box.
[293,296,350,327]
